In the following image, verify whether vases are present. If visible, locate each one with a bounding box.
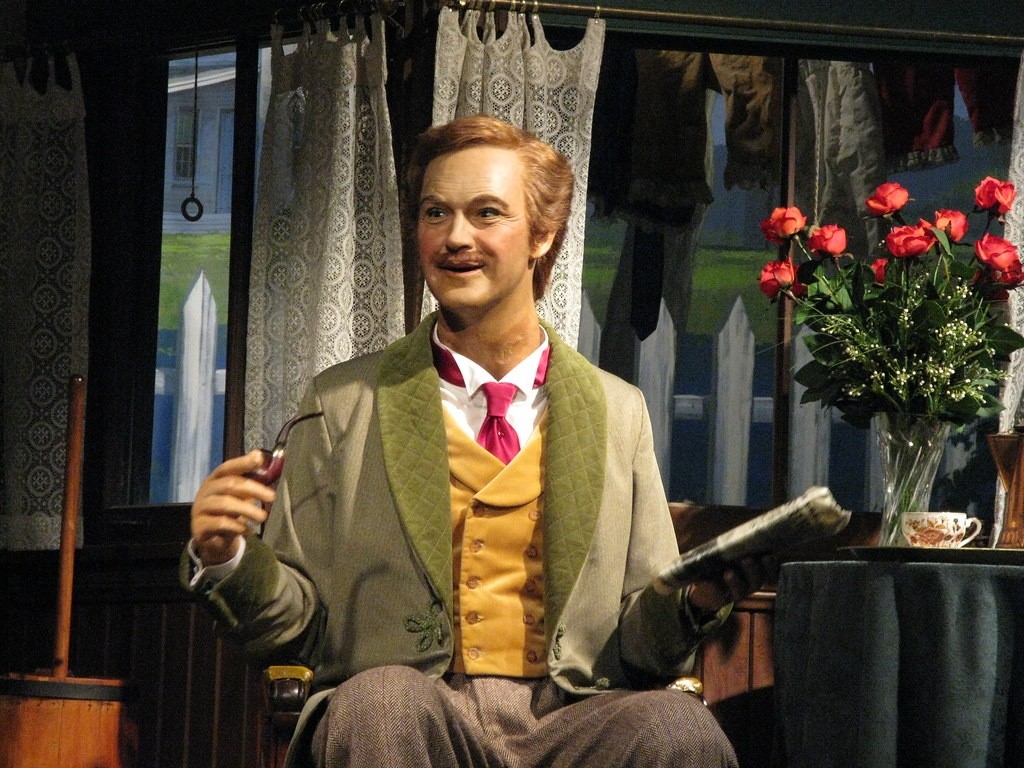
[867,412,950,548]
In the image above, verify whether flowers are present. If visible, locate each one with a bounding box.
[752,174,1024,538]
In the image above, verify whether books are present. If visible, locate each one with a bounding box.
[650,486,852,597]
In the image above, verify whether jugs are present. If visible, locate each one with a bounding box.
[990,422,1024,549]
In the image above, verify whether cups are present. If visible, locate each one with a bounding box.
[901,511,982,549]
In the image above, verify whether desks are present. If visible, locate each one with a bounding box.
[769,544,1024,768]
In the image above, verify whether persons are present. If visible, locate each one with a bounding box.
[181,114,757,768]
